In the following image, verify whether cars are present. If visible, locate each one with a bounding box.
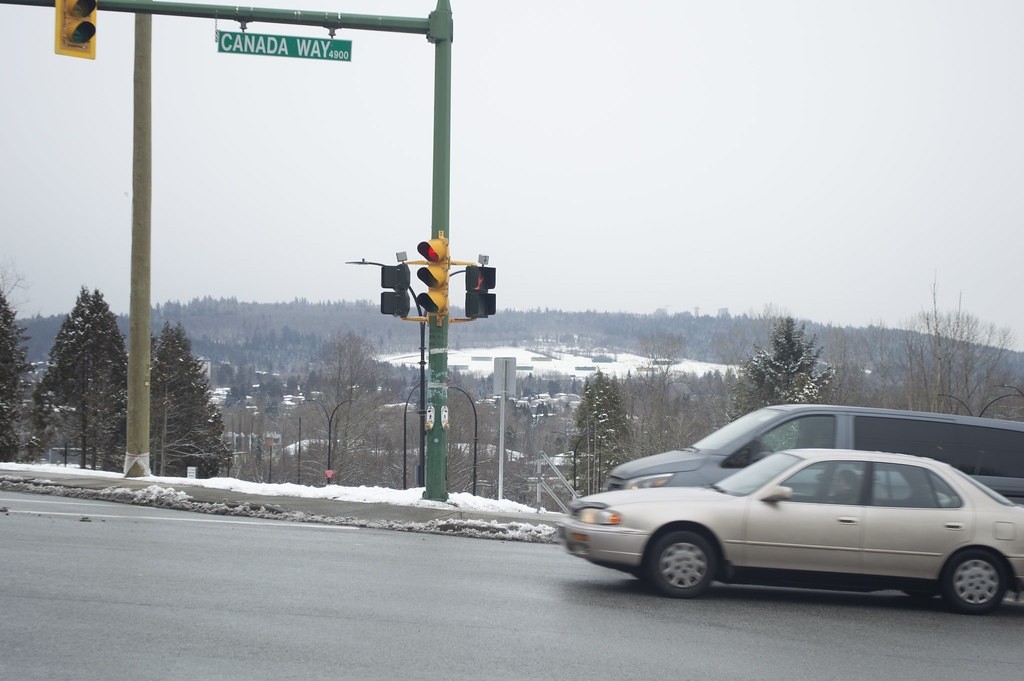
[556,447,1024,615]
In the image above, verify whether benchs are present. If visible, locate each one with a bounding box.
[935,491,951,508]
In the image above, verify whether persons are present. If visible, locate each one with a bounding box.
[817,471,862,505]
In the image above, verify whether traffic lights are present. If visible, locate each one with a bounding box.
[381,263,410,318]
[53,0,97,60]
[417,238,450,317]
[464,265,497,318]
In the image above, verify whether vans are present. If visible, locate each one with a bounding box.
[604,402,1024,505]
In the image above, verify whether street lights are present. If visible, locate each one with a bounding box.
[301,398,358,485]
